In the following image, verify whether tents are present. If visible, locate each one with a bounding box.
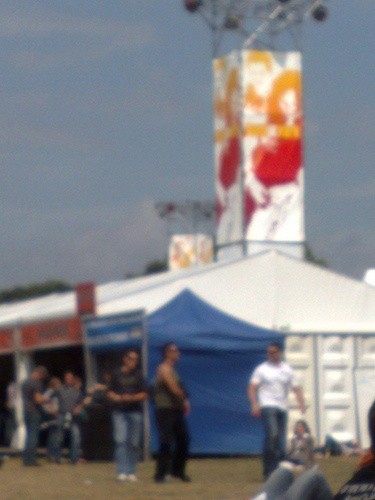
[81,288,286,453]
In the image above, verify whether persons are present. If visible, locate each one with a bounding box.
[103,350,149,482]
[152,342,192,483]
[287,420,314,467]
[21,366,82,466]
[247,340,306,479]
[253,400,375,500]
